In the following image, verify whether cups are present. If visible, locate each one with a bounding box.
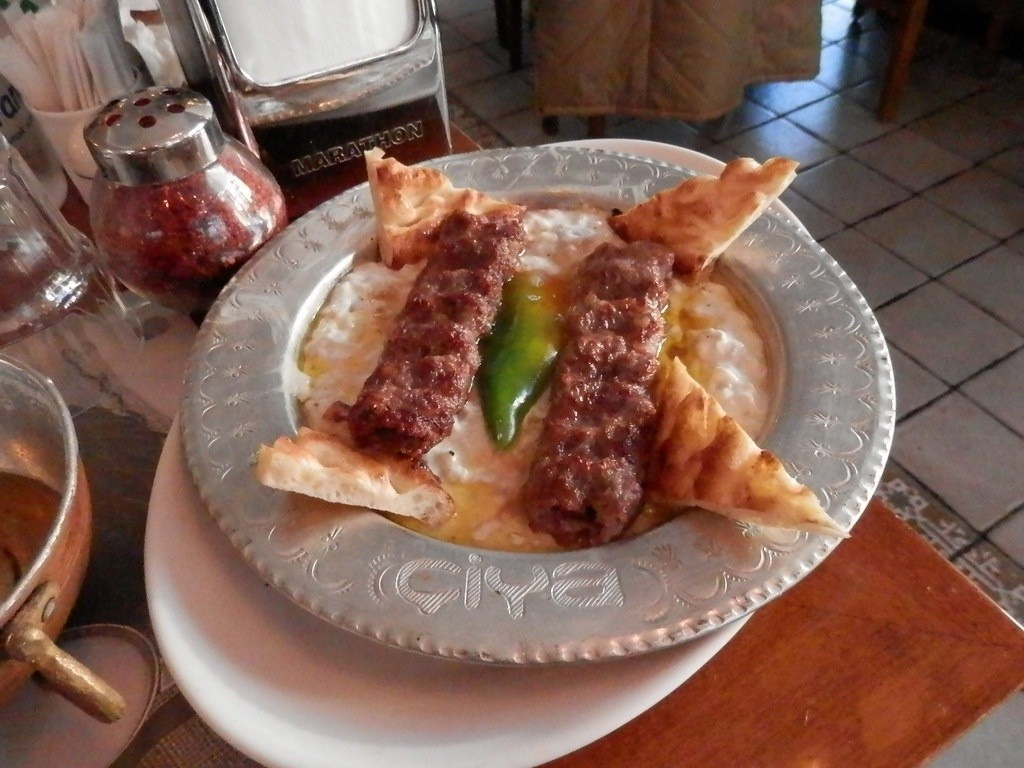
[21,65,147,207]
[0,129,138,420]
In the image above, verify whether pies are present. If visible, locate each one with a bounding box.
[261,143,850,541]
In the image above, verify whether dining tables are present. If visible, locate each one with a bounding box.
[0,119,1024,768]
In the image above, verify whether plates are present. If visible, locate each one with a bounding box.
[178,143,902,663]
[143,136,813,765]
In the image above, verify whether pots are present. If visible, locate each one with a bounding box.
[0,347,126,727]
